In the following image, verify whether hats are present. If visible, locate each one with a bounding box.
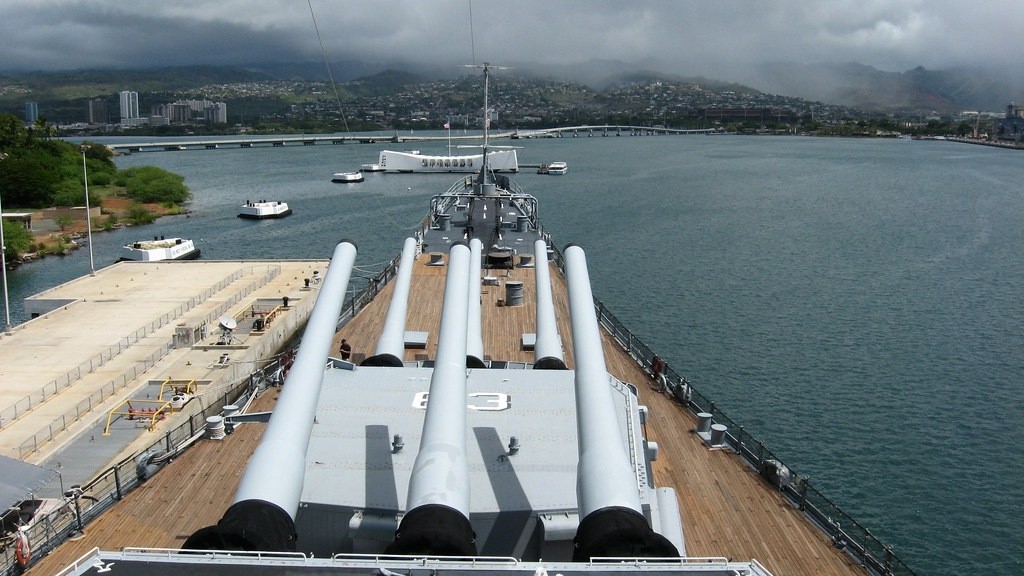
[342,339,346,343]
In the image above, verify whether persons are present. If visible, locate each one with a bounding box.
[283,349,294,380]
[339,339,351,361]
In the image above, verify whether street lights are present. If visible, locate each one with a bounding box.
[0,153,12,333]
[77,143,95,278]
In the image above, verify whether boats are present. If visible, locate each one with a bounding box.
[537,161,568,175]
[329,171,365,183]
[120,235,202,261]
[237,198,293,220]
[359,147,520,173]
